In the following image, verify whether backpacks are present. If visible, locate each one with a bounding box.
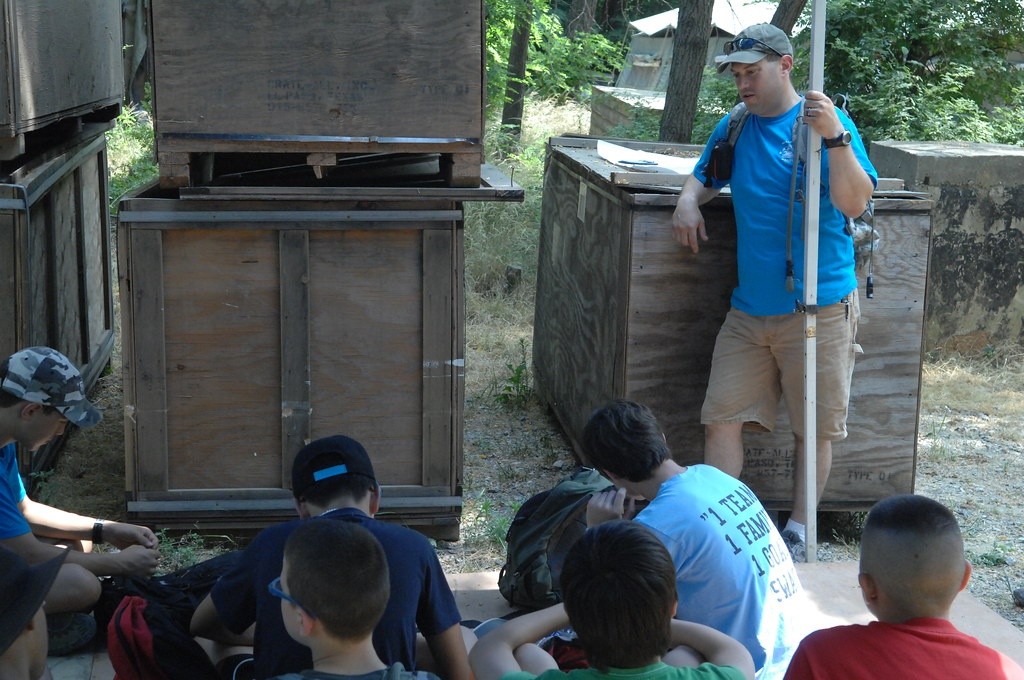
[497,464,619,610]
[94,550,245,646]
[107,596,221,680]
[702,92,880,280]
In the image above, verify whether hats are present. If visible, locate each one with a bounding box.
[716,22,794,74]
[0,543,72,658]
[291,434,377,500]
[1,347,104,431]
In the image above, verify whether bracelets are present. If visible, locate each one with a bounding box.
[92,518,104,545]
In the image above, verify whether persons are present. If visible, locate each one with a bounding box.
[189,435,476,680]
[0,344,161,680]
[671,22,878,563]
[468,519,756,680]
[263,519,442,680]
[580,399,804,680]
[783,495,1007,680]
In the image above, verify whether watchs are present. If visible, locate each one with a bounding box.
[823,131,852,149]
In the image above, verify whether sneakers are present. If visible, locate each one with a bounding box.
[44,611,98,657]
[780,530,808,562]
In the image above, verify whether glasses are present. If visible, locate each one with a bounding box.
[268,577,318,622]
[723,38,782,59]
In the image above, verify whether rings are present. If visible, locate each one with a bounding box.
[807,108,811,116]
